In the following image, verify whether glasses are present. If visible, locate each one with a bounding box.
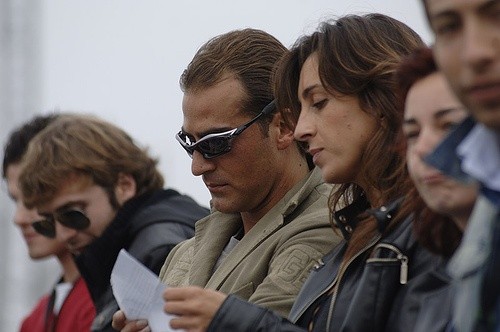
[175,99,279,159]
[32,189,111,238]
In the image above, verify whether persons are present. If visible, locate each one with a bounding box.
[112,14,455,332]
[387,0,500,332]
[398,48,476,260]
[0,113,211,332]
[158,28,353,319]
